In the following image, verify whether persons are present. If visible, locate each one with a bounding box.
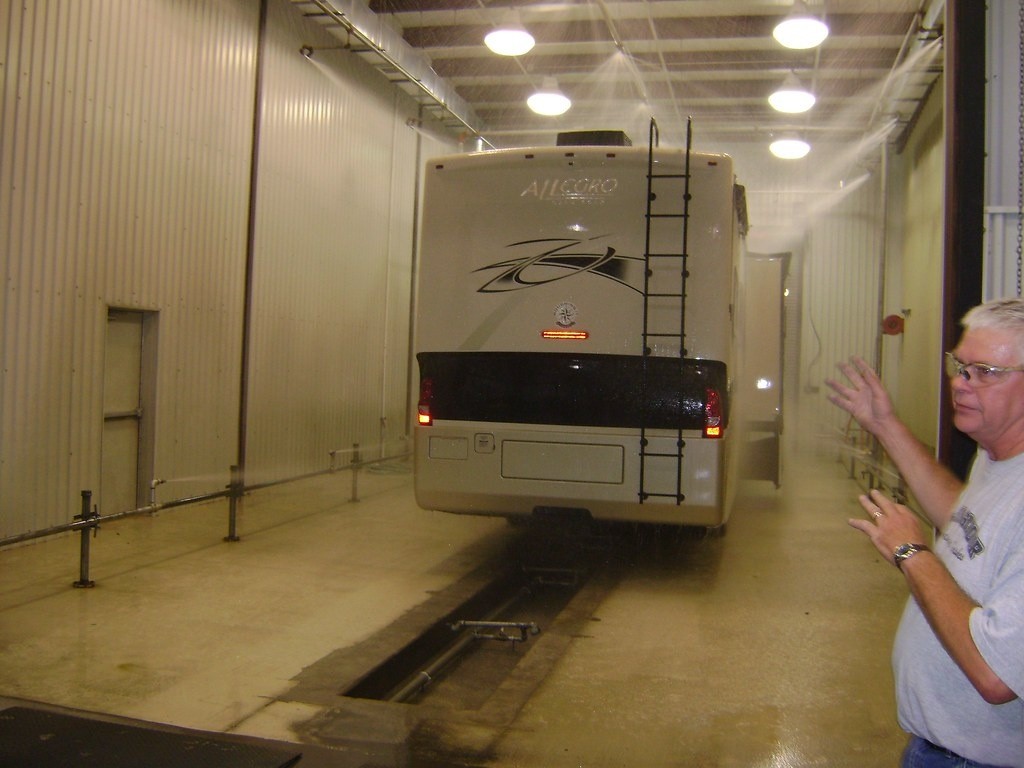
[823,299,1024,768]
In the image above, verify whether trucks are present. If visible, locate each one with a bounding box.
[412,121,789,532]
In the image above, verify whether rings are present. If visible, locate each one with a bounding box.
[871,511,884,521]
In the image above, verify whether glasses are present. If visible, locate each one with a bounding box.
[944,351,1024,388]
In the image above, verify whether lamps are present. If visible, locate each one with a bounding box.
[485,1,535,56]
[768,67,815,115]
[526,70,571,117]
[769,118,811,160]
[773,0,828,50]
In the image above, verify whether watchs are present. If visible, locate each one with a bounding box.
[892,542,934,575]
